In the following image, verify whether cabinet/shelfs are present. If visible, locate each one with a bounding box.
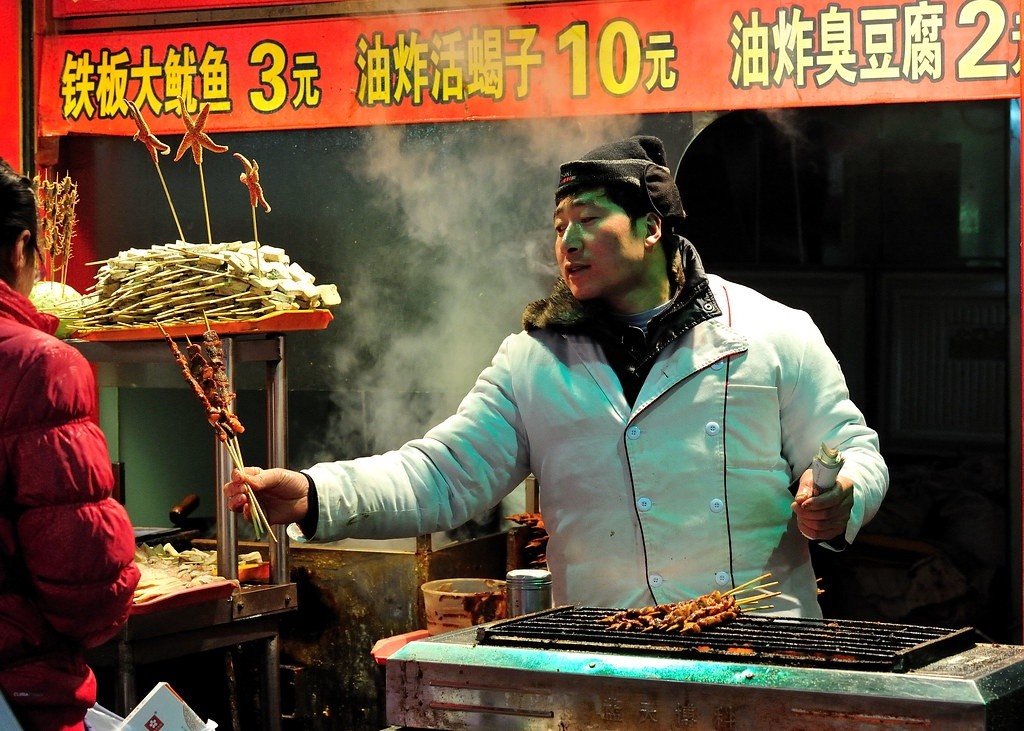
[64,336,299,731]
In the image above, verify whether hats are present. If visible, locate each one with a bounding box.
[554,134,670,199]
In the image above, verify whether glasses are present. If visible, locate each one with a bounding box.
[33,241,47,285]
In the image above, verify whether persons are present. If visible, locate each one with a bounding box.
[0,157,141,730]
[219,135,888,618]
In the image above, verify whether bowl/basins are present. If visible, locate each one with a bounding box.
[421,578,508,636]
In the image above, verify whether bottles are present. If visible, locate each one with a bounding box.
[506,569,553,619]
